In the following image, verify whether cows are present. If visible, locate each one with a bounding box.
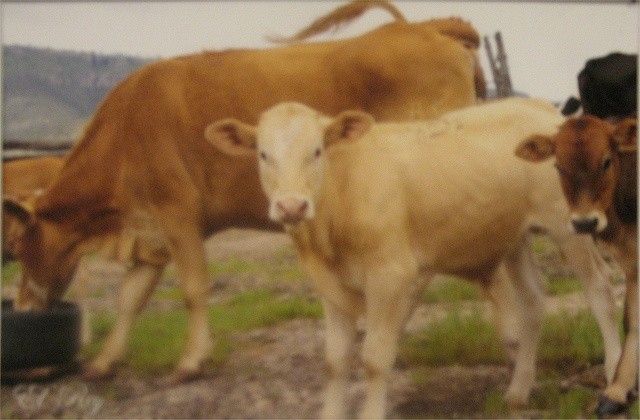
[512,117,640,418]
[203,97,623,419]
[0,0,491,388]
